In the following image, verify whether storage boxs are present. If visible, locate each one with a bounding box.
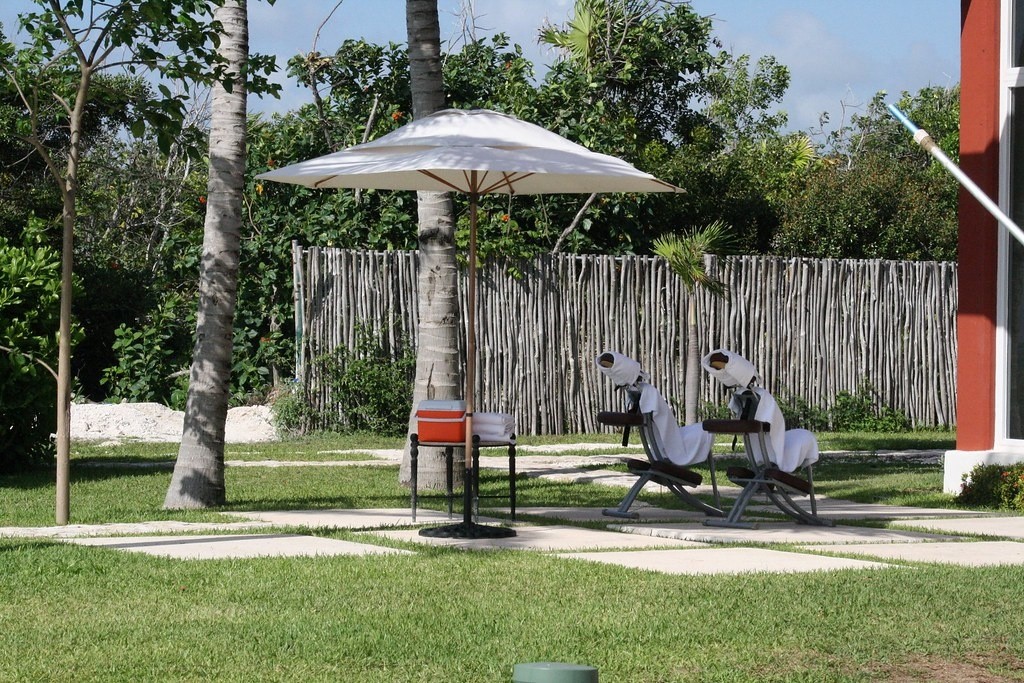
[412,400,466,442]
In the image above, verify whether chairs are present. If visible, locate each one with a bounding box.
[596,351,725,519]
[701,349,836,529]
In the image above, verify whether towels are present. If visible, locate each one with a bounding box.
[727,386,820,473]
[627,382,716,465]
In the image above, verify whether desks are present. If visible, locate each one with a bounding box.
[409,432,517,525]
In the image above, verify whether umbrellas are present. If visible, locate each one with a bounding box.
[254,107,688,522]
